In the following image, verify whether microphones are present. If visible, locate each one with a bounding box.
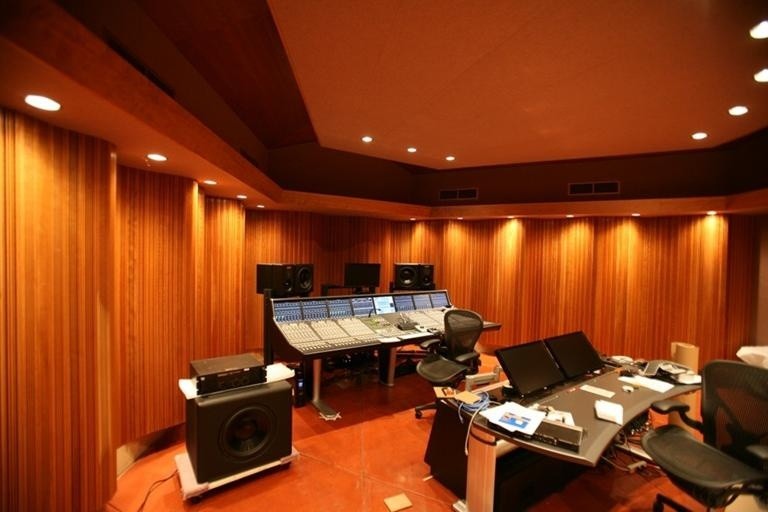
[368,308,382,317]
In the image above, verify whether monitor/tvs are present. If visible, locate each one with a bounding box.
[495,340,568,398]
[344,263,379,287]
[544,331,605,379]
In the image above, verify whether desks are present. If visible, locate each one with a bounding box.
[435,360,702,511]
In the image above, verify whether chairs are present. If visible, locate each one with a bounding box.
[414,309,485,419]
[643,360,766,512]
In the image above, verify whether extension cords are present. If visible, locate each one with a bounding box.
[628,461,648,475]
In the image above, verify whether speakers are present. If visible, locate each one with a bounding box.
[256,263,314,297]
[185,380,291,483]
[391,265,435,290]
